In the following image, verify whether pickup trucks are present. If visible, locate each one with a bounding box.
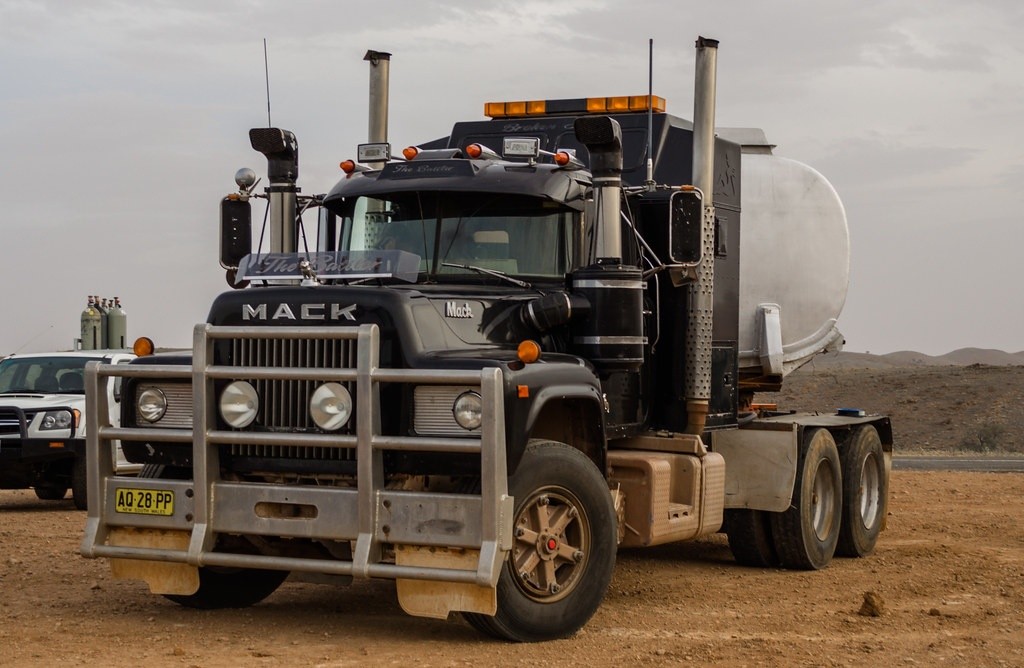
[0,339,138,510]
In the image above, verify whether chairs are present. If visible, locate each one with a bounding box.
[59,372,84,392]
[33,375,59,391]
[378,223,417,255]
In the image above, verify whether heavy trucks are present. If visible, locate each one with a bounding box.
[79,36,895,643]
